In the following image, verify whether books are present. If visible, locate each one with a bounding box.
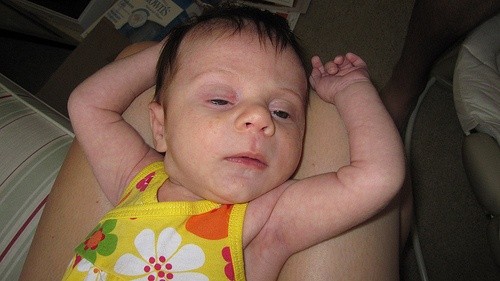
[79,0,311,41]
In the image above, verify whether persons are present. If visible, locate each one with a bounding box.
[61,5,406,281]
